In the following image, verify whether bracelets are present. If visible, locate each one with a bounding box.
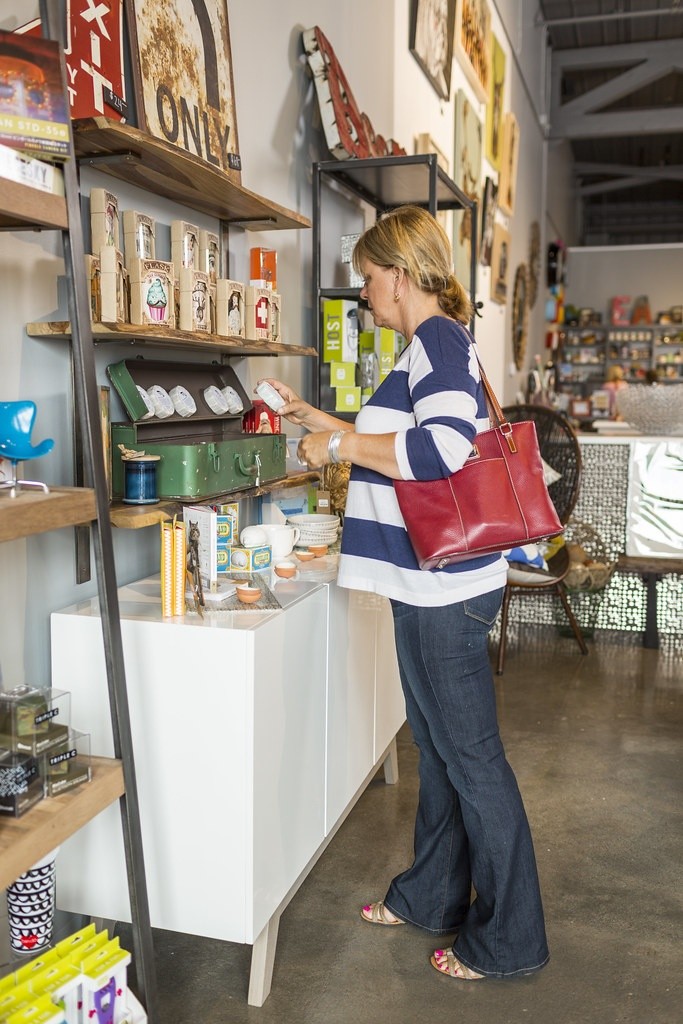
[328,430,345,463]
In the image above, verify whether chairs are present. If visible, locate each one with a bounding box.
[495,405,589,677]
[0,401,55,498]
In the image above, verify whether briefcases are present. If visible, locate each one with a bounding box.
[104,354,288,503]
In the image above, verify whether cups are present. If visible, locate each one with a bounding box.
[254,524,299,559]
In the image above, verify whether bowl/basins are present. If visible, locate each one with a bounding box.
[235,545,328,604]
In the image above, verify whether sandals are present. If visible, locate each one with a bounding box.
[360,900,407,925]
[430,946,532,980]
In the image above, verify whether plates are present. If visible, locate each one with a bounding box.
[286,513,341,547]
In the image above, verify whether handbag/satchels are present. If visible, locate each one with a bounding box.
[393,318,564,571]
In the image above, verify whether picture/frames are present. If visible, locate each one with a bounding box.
[409,0,521,303]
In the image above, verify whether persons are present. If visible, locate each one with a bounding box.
[253,204,549,979]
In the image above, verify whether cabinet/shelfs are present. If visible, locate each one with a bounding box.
[561,326,683,400]
[28,115,321,586]
[312,152,480,425]
[49,553,408,1006]
[0,0,165,1024]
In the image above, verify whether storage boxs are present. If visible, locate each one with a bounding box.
[0,686,93,820]
[216,502,272,574]
[323,233,407,412]
[0,922,131,1024]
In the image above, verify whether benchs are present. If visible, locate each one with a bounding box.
[615,557,683,649]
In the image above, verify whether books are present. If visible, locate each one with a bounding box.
[185,576,252,605]
[182,505,218,591]
[0,29,72,198]
